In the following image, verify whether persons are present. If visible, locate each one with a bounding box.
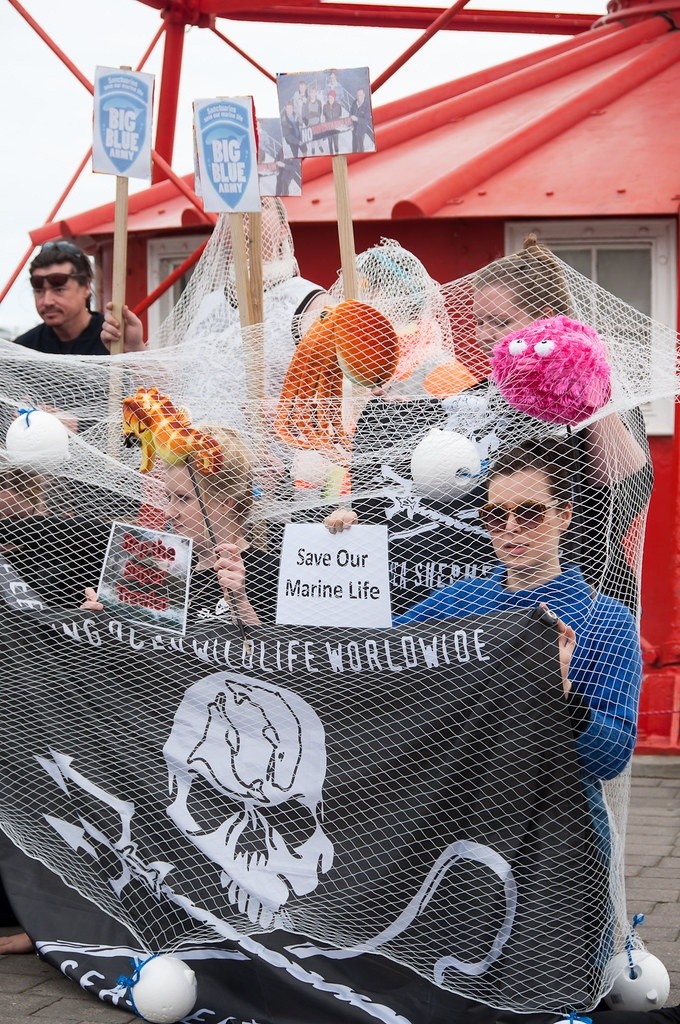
[275,72,368,196]
[0,195,654,1005]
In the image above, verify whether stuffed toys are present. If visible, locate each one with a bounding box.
[272,299,400,449]
[122,386,223,474]
[490,315,612,425]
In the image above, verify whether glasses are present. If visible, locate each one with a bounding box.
[479,499,562,532]
[29,273,86,289]
[42,241,84,257]
